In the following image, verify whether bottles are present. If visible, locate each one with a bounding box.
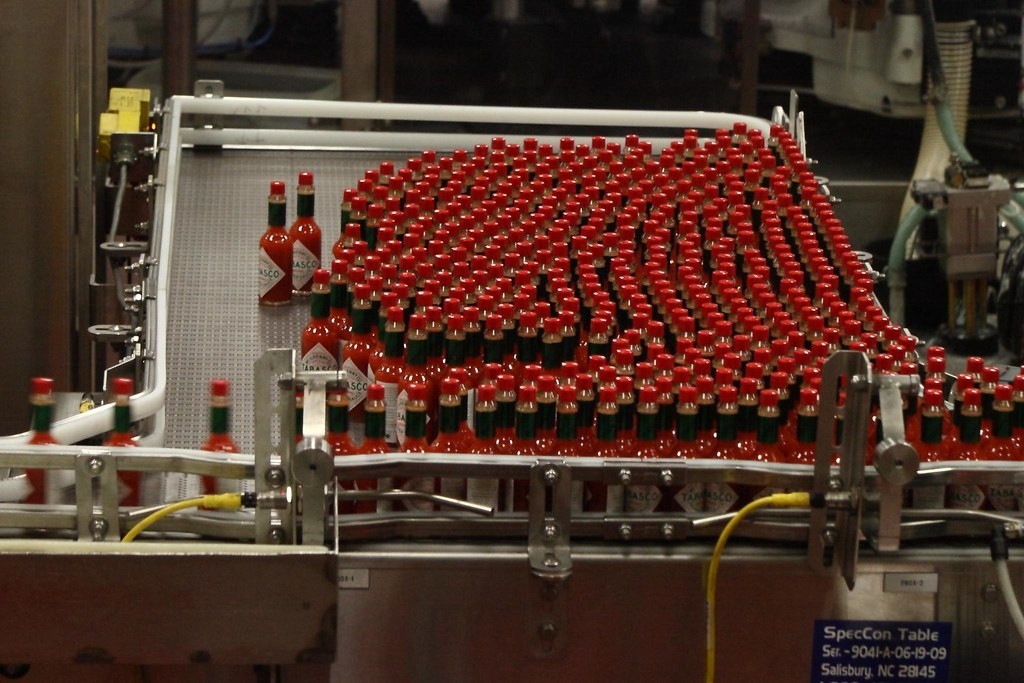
[21,378,64,503]
[104,379,141,506]
[259,122,1023,513]
[198,378,240,512]
